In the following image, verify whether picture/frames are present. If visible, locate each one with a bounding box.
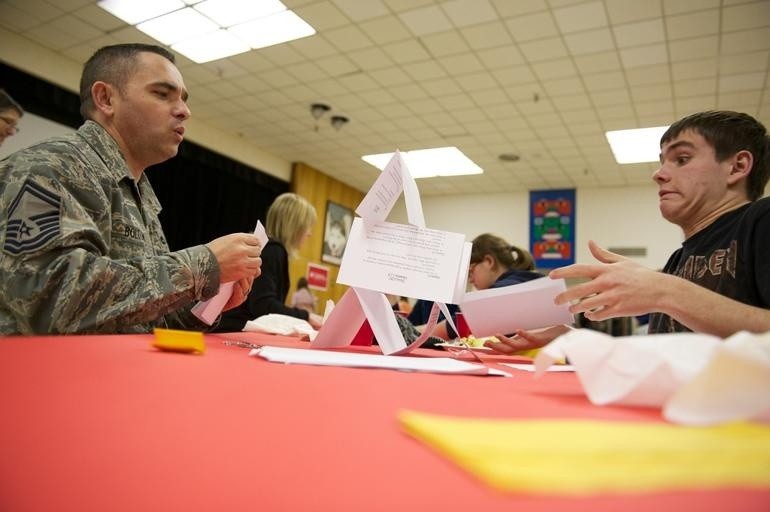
[321,200,354,267]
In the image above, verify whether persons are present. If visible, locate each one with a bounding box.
[0,43,262,337]
[289,277,315,313]
[0,90,23,146]
[481,107,770,356]
[413,233,548,341]
[205,192,323,334]
[391,295,413,313]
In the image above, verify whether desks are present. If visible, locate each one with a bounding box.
[0,329,770,512]
